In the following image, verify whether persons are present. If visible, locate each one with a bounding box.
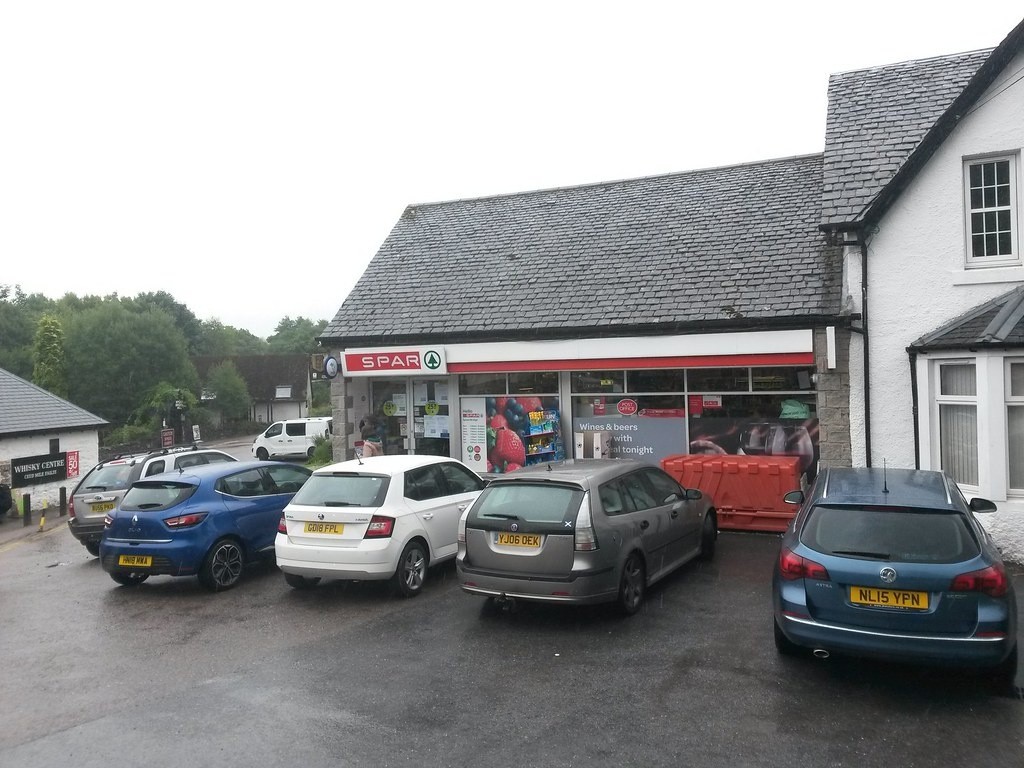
[354,412,388,459]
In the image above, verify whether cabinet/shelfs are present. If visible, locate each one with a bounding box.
[522,409,565,467]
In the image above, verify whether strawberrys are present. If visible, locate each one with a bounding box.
[485,395,543,472]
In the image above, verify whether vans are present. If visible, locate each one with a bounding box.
[249,415,334,462]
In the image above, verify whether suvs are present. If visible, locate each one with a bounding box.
[769,466,1019,682]
[271,454,505,598]
[455,457,719,617]
[97,461,313,593]
[67,444,251,556]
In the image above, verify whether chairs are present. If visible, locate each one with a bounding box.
[241,472,261,496]
[408,469,436,498]
